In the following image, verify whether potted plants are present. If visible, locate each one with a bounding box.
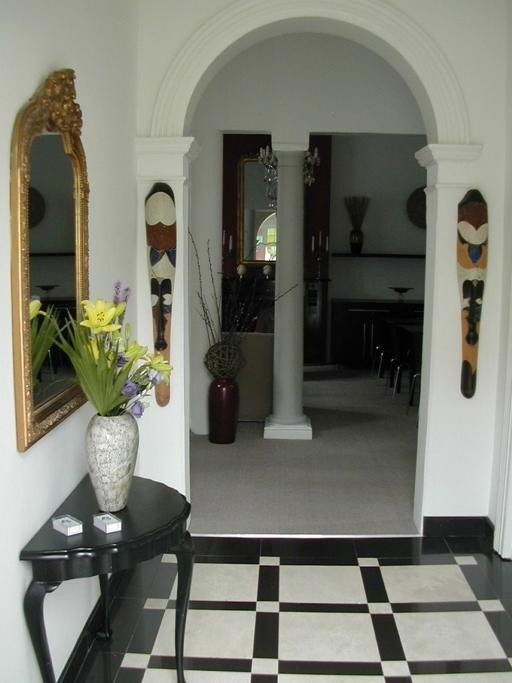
[341,194,372,256]
[202,339,250,446]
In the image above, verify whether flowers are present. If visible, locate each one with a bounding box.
[56,296,174,416]
[30,290,62,383]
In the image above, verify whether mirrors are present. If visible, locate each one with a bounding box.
[11,65,92,454]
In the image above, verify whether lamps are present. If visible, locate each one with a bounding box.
[304,147,320,187]
[258,145,278,210]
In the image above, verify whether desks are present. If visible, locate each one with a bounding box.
[20,472,197,682]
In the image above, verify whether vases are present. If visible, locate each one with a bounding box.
[83,407,140,513]
